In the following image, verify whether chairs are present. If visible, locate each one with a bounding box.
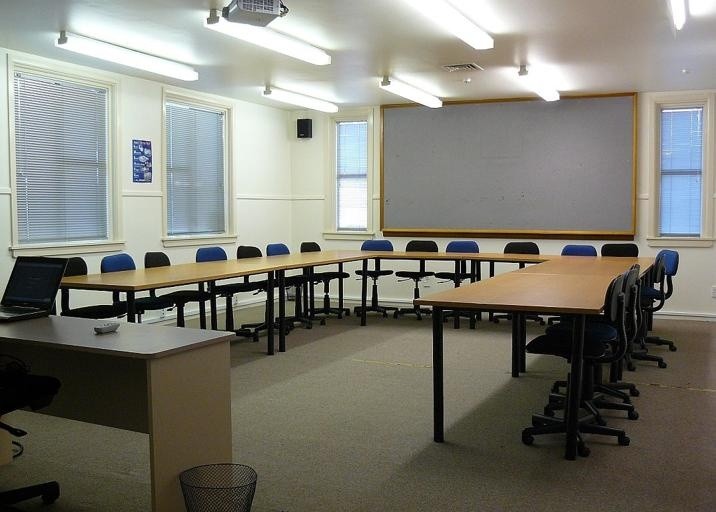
[0,418,63,506]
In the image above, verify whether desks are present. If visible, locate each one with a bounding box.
[1,312,237,512]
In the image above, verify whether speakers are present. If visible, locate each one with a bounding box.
[297,119,312,138]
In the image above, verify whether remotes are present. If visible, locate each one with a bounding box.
[93,321,120,334]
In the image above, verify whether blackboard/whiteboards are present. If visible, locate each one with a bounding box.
[379,90,639,241]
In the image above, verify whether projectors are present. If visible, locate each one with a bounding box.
[221,0,282,28]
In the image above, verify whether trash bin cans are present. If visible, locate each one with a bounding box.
[285,285,297,301]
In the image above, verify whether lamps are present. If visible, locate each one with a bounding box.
[379,75,442,112]
[261,83,339,117]
[396,0,494,72]
[55,29,198,83]
[513,60,559,103]
[202,8,332,69]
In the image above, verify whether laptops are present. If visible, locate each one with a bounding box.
[0,256,69,323]
